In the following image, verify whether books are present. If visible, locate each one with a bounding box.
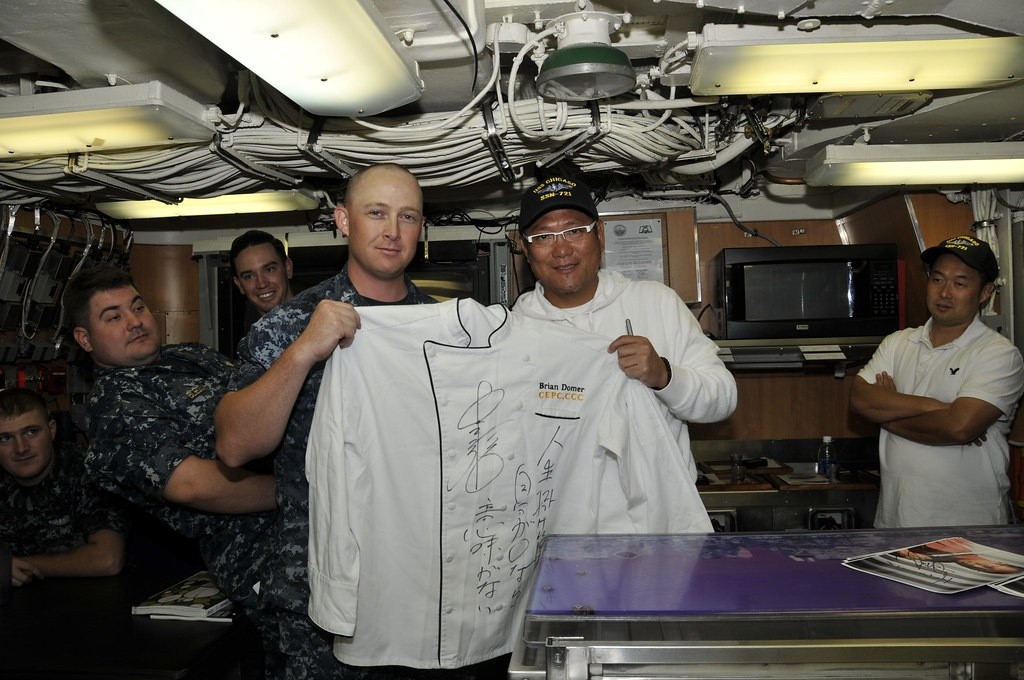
[131,569,244,624]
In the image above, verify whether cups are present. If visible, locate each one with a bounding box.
[826,464,840,480]
[731,454,743,480]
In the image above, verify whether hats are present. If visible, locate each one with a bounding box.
[920,235,999,296]
[517,176,600,238]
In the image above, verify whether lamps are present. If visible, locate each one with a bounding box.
[154,1,424,118]
[687,23,1024,96]
[94,188,320,220]
[804,126,1024,187]
[536,10,638,101]
[0,81,217,160]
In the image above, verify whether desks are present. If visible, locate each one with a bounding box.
[506,526,1024,680]
[0,575,263,680]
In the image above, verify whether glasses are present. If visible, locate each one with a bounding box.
[522,220,597,248]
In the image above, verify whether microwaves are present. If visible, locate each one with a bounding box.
[709,243,899,340]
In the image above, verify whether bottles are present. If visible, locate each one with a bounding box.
[819,436,835,474]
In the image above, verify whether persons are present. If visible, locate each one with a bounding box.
[63,264,284,680]
[888,538,1024,574]
[228,229,299,343]
[848,234,1024,528]
[214,163,479,680]
[509,172,739,534]
[0,385,130,589]
